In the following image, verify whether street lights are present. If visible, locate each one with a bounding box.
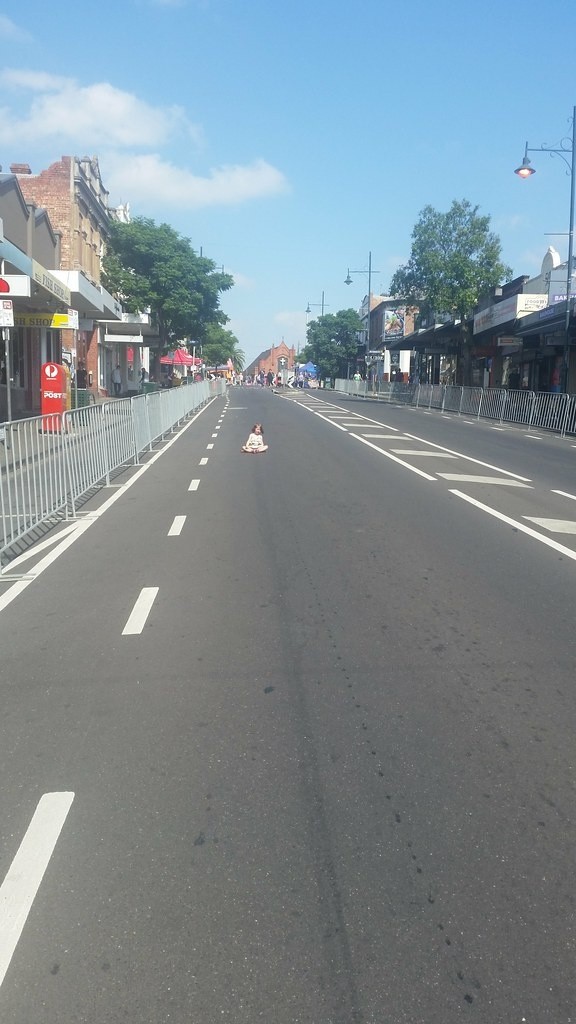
[513,143,575,396]
[345,270,372,392]
[305,303,324,317]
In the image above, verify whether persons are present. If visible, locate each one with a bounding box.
[112,365,148,395]
[395,368,420,387]
[257,367,273,387]
[507,367,520,401]
[242,423,268,453]
[71,362,86,389]
[353,371,364,392]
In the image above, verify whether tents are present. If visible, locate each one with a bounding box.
[296,361,317,389]
[161,349,203,385]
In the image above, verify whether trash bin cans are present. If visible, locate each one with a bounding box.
[181,376,193,385]
[141,382,158,394]
[70,388,91,427]
